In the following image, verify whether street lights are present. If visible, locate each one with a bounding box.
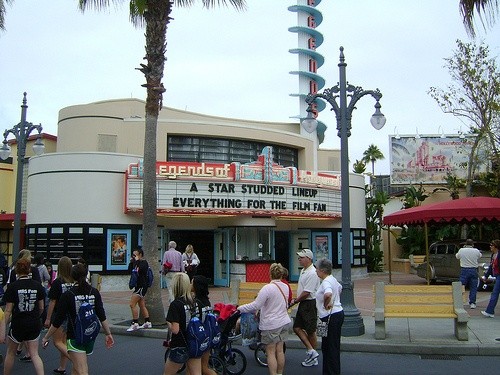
[300,44,387,339]
[0,91,45,285]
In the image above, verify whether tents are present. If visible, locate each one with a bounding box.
[384,197,500,285]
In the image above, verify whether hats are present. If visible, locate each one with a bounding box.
[296,249,313,260]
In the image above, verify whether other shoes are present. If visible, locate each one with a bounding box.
[53,368,66,375]
[471,303,476,308]
[19,356,33,361]
[15,349,21,355]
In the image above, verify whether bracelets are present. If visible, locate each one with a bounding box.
[294,298,298,304]
[105,332,111,335]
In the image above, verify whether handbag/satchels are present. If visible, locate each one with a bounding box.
[316,322,328,336]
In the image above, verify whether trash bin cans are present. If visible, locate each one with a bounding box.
[90,274,101,291]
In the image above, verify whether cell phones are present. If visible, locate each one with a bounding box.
[44,342,49,347]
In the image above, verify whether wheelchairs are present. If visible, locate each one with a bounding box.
[164,309,248,375]
[254,299,301,368]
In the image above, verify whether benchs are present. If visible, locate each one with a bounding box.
[409,255,425,274]
[373,281,468,341]
[237,279,298,334]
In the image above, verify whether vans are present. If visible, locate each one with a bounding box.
[424,239,499,290]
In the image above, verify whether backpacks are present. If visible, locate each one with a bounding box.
[176,299,210,358]
[184,254,195,271]
[68,287,101,344]
[196,299,222,347]
[146,268,153,287]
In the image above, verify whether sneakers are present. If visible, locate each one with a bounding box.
[127,323,140,332]
[305,350,319,363]
[139,321,152,328]
[481,310,495,318]
[301,358,318,367]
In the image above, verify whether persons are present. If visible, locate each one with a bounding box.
[480,240,500,317]
[127,246,152,331]
[280,268,292,375]
[455,239,482,309]
[315,258,344,375]
[162,241,185,304]
[163,272,218,375]
[0,249,114,375]
[237,263,292,375]
[290,249,320,366]
[182,245,200,284]
